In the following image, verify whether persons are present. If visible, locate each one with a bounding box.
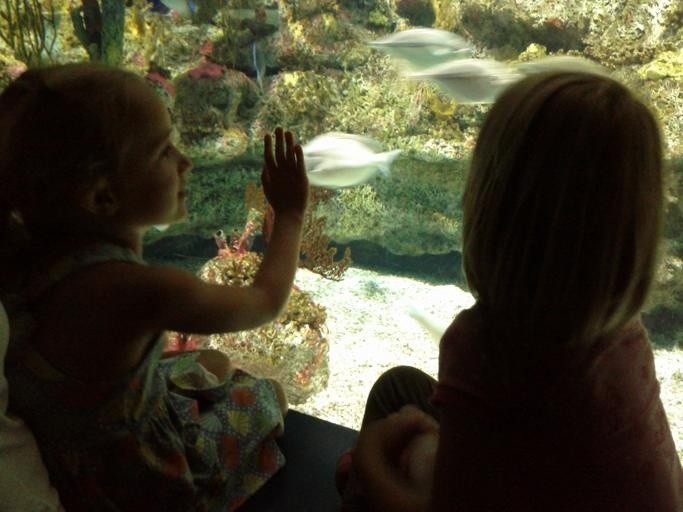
[0,61,308,512]
[336,71,683,509]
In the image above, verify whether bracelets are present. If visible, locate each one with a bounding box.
[270,210,305,230]
